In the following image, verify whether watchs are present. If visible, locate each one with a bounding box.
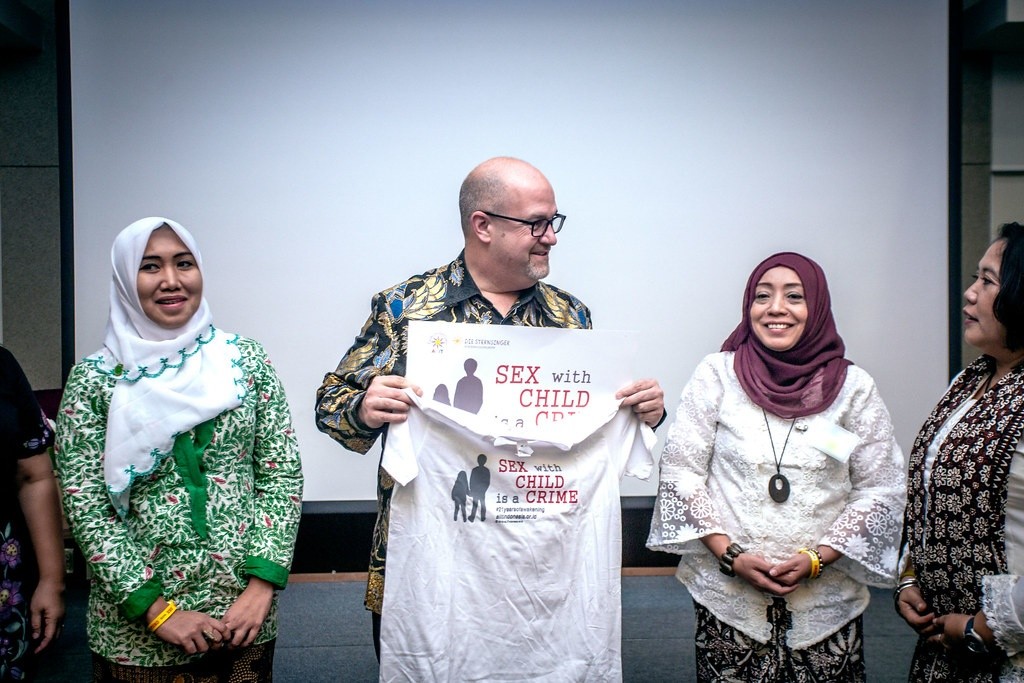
[963,617,989,654]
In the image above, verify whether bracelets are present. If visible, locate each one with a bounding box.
[801,547,823,579]
[719,543,743,577]
[893,570,919,599]
[147,599,178,632]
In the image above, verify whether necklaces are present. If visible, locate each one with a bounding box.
[763,409,796,503]
[985,375,993,393]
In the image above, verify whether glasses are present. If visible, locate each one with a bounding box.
[479,210,566,237]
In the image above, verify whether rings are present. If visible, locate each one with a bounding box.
[937,634,942,644]
[204,630,214,639]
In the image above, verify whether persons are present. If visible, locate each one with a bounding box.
[896,223,1024,683]
[54,216,304,683]
[645,252,907,683]
[0,346,66,683]
[315,157,667,665]
